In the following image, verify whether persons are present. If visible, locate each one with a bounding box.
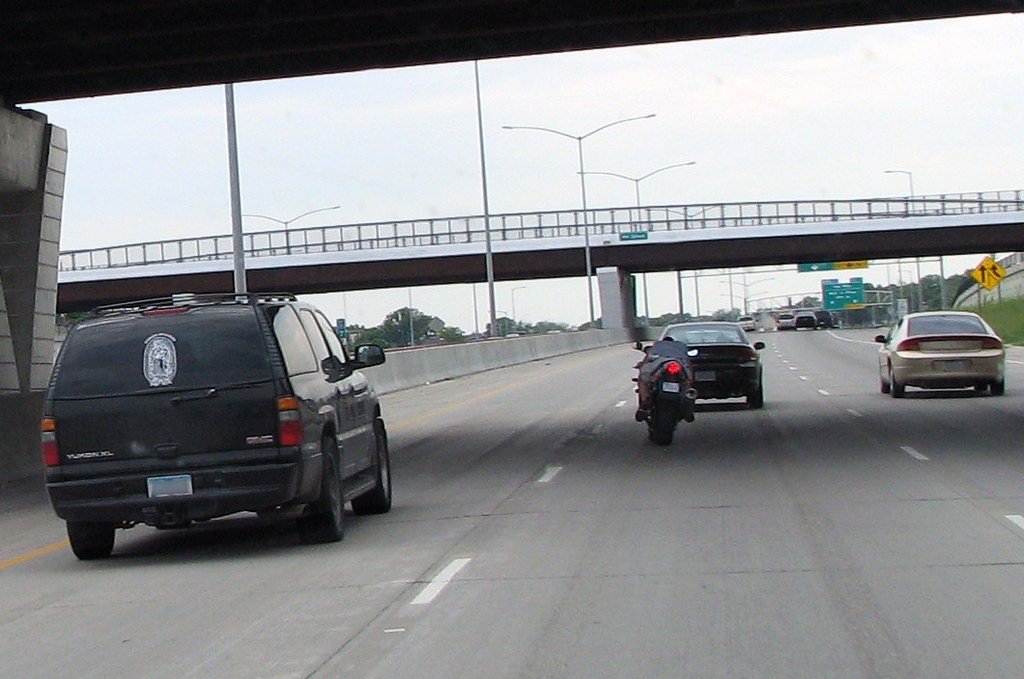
[636,336,695,423]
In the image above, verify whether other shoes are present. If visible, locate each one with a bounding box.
[684,411,694,423]
[636,401,649,422]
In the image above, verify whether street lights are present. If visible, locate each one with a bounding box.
[884,170,915,212]
[241,206,341,255]
[576,161,696,232]
[502,114,658,322]
[720,277,775,316]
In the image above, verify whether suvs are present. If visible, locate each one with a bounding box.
[40,291,392,559]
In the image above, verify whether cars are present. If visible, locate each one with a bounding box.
[547,330,561,335]
[794,311,817,331]
[736,316,758,330]
[659,321,765,409]
[775,312,797,331]
[874,310,1007,399]
[814,311,833,330]
[486,334,520,341]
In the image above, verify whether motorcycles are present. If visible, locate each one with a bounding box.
[631,341,699,447]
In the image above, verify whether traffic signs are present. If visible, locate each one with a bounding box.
[823,282,866,311]
